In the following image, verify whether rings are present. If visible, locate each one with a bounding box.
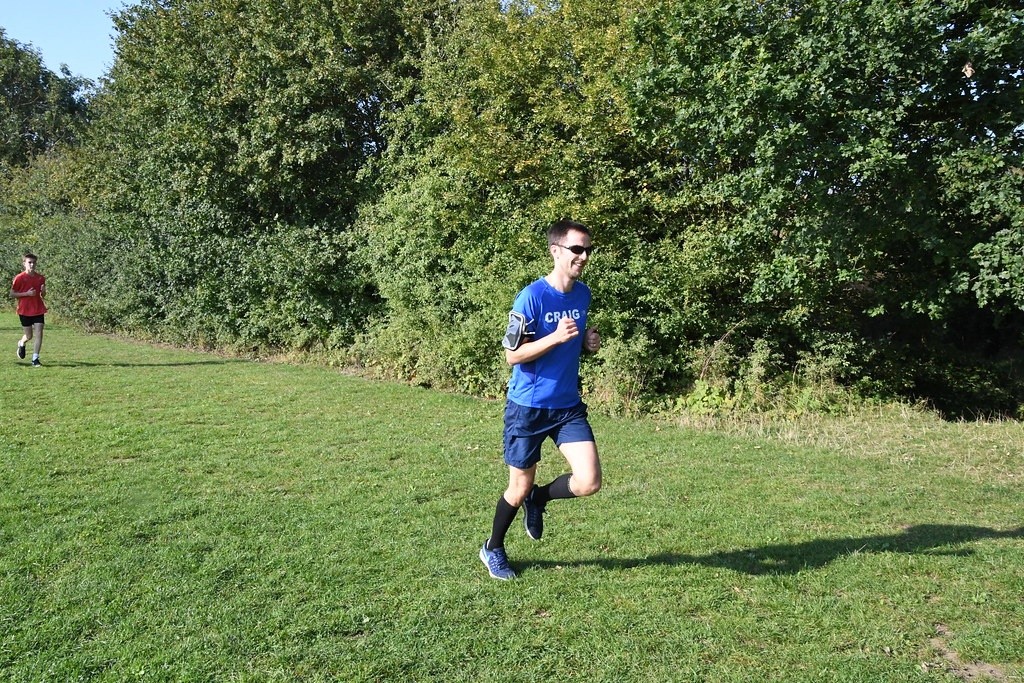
[594,344,595,347]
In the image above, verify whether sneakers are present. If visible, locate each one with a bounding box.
[16,340,26,359]
[521,484,547,540]
[31,357,42,367]
[479,538,517,582]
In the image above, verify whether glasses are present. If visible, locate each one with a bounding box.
[559,245,593,256]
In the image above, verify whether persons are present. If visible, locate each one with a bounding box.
[9,254,46,367]
[478,218,601,583]
